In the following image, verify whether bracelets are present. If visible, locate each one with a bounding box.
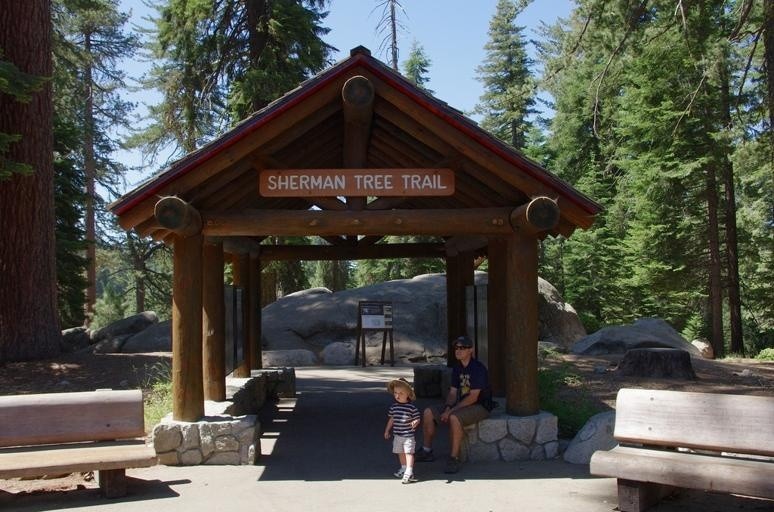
[445,403,453,409]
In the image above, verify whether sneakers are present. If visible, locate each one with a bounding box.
[394,468,414,483]
[446,456,459,473]
[415,447,434,462]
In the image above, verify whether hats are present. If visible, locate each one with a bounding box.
[389,378,416,403]
[452,336,472,345]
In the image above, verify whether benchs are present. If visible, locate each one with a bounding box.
[589,388,773,511]
[0,388,158,498]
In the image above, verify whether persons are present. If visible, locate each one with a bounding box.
[414,333,495,475]
[381,376,421,486]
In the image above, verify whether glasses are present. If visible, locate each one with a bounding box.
[455,345,470,350]
